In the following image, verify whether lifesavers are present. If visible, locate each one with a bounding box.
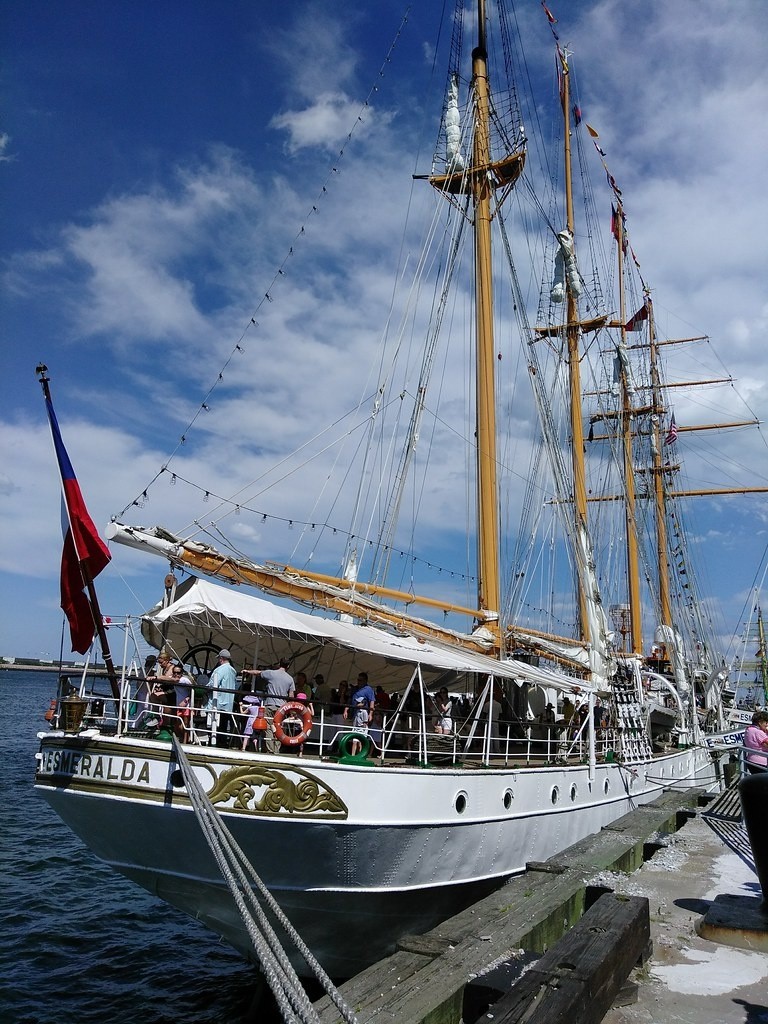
[270,700,315,746]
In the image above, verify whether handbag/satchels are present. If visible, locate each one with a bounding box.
[207,712,220,728]
[433,725,442,734]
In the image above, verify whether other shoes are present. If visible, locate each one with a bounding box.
[153,729,160,736]
[241,748,245,753]
[146,718,158,726]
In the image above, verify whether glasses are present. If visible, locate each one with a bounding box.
[173,671,181,674]
[339,682,345,687]
[440,691,446,694]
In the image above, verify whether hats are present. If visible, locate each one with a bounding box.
[354,696,367,704]
[216,649,231,659]
[146,655,157,662]
[296,693,307,700]
[545,704,555,708]
[413,678,419,684]
[562,698,570,703]
[313,674,323,679]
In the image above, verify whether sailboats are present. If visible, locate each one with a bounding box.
[33,0,768,980]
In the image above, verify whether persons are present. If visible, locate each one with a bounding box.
[743,709,768,774]
[130,648,193,745]
[539,697,610,752]
[404,677,462,760]
[207,649,239,749]
[484,694,508,756]
[240,656,295,755]
[239,694,261,753]
[296,672,400,756]
[665,666,675,676]
